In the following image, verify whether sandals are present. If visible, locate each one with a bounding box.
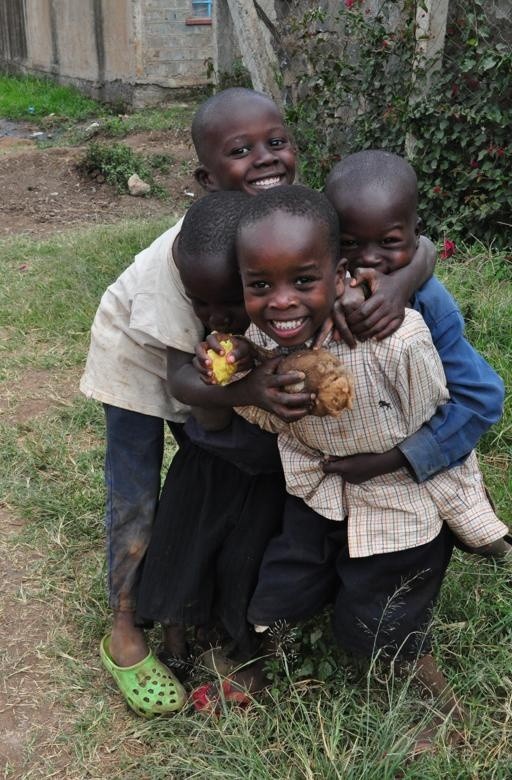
[98,630,189,720]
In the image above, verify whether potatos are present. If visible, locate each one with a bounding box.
[278,348,355,418]
[206,331,237,387]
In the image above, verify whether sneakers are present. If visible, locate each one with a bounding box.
[202,645,272,703]
[384,652,464,720]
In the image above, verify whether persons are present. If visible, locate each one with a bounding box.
[69,82,511,763]
